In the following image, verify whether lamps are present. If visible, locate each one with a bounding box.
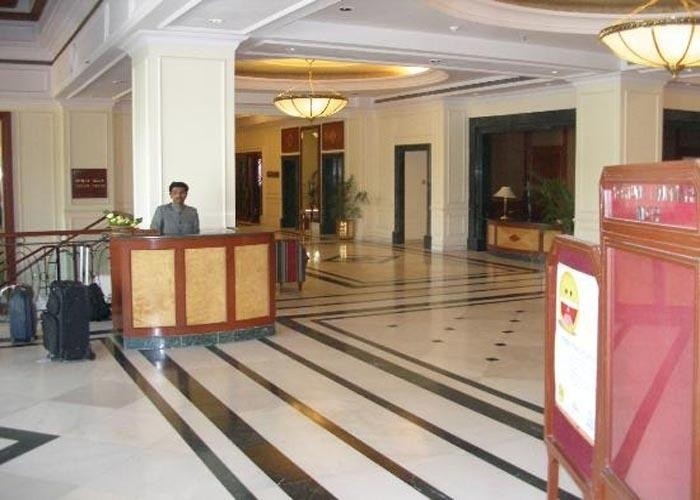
[597,0,700,77]
[494,185,517,219]
[272,57,348,122]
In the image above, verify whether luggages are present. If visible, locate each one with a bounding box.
[7,283,37,347]
[39,279,96,362]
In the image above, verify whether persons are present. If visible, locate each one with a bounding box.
[150,182,199,235]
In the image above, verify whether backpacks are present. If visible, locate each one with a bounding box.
[84,281,111,323]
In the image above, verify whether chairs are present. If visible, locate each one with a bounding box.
[276,238,310,291]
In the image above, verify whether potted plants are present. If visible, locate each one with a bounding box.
[327,174,370,240]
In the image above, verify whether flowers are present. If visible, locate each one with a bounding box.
[103,209,143,227]
